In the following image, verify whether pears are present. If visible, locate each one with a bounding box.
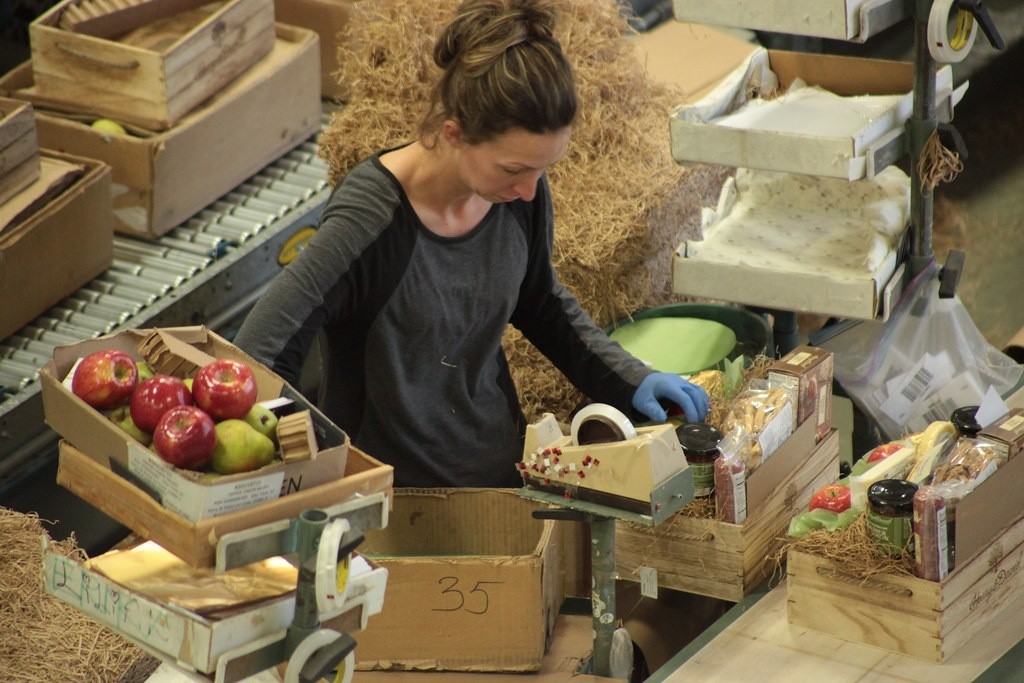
[109,361,283,480]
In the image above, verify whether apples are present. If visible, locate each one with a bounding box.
[130,374,193,433]
[191,358,258,423]
[72,349,139,411]
[153,404,217,469]
[808,484,852,514]
[866,443,904,463]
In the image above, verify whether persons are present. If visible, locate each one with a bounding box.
[232,0,707,488]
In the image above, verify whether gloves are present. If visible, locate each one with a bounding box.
[634,371,713,428]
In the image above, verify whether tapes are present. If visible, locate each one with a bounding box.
[570,403,638,447]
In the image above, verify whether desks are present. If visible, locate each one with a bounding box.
[639,572,1024,683]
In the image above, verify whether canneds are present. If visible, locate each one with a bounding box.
[674,423,723,499]
[865,478,920,558]
[951,405,985,440]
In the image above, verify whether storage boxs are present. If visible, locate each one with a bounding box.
[356,487,587,676]
[672,0,864,40]
[785,495,1024,665]
[43,542,388,672]
[37,323,350,517]
[768,344,834,447]
[0,0,357,346]
[613,425,841,602]
[673,216,910,325]
[53,429,394,568]
[671,46,959,185]
[975,406,1024,464]
